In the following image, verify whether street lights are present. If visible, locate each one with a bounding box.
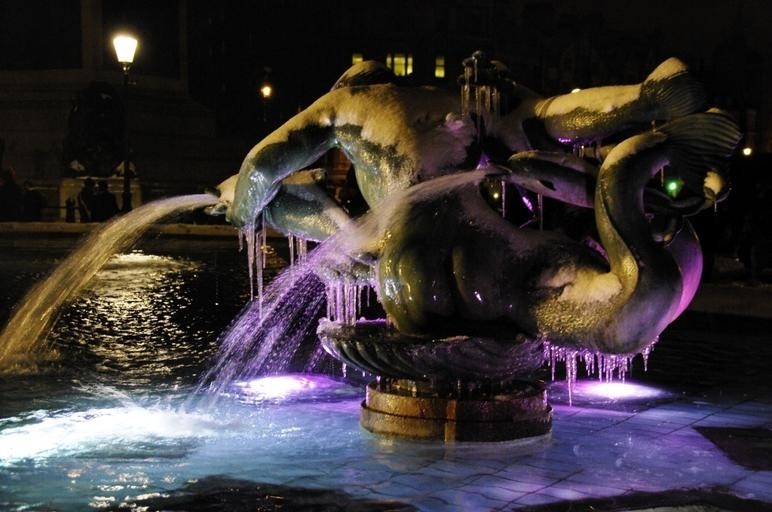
[261,85,271,136]
[111,29,138,212]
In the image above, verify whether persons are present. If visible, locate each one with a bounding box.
[91,178,120,223]
[81,177,97,213]
[336,165,369,218]
